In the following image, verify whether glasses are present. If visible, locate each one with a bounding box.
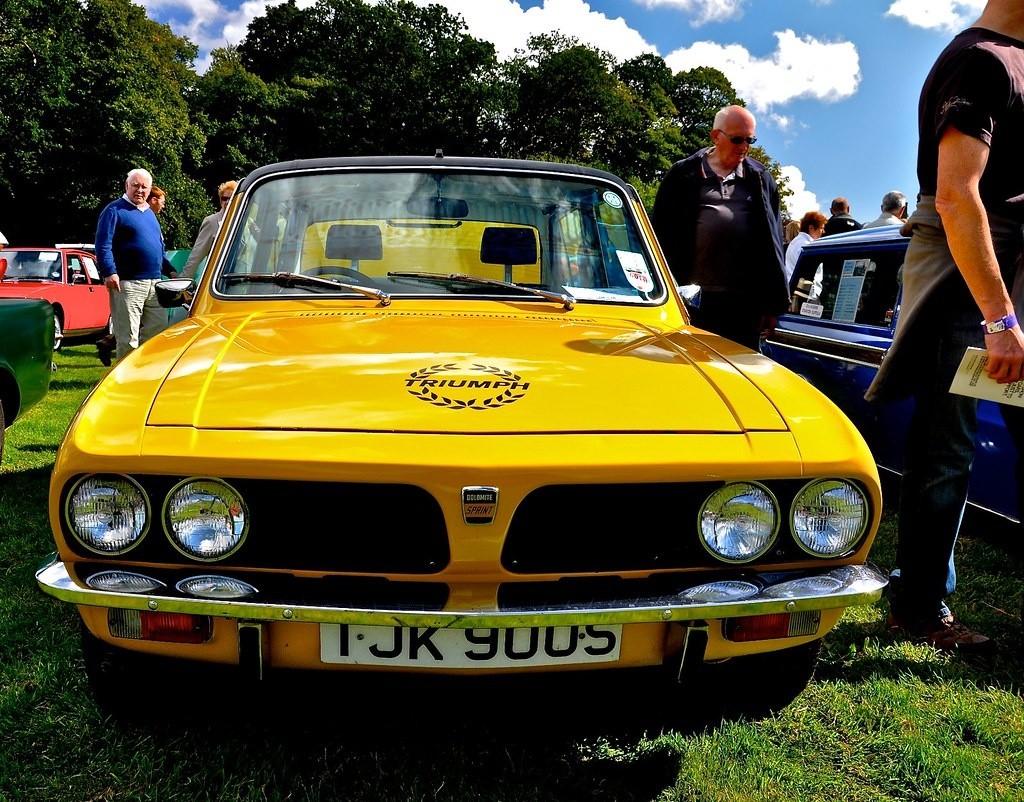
[718,128,756,144]
[221,196,231,202]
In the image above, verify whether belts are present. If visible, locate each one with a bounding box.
[689,282,727,292]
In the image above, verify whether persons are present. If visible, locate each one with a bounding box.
[177,181,262,278]
[883,2,1023,664]
[782,192,908,310]
[652,105,792,350]
[95,168,179,367]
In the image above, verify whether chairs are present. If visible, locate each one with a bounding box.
[325,224,382,281]
[480,227,551,300]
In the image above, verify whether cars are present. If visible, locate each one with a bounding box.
[766,224,1023,535]
[0,249,116,351]
[35,147,889,725]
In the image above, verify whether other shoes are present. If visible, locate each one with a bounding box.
[97,334,117,366]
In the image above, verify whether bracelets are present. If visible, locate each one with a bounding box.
[981,313,1017,335]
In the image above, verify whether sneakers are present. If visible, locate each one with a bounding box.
[885,601,997,666]
